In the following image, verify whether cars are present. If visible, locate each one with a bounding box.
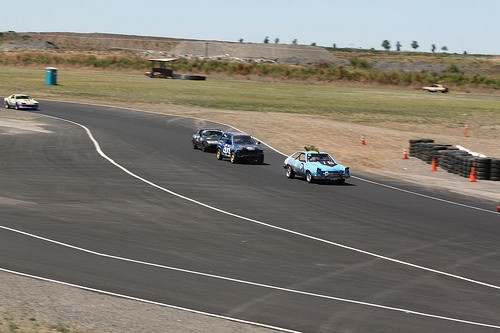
[421,83,448,93]
[191,128,227,152]
[3,93,39,110]
[282,145,350,185]
[215,131,265,165]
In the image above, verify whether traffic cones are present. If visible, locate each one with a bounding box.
[360,134,366,145]
[402,146,408,159]
[431,159,437,171]
[468,167,476,182]
